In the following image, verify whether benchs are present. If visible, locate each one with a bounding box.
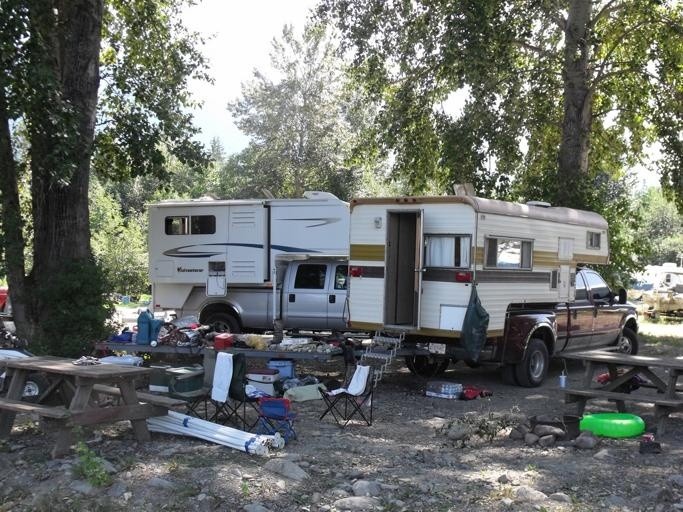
[0,399,72,459]
[92,383,187,443]
[561,381,683,441]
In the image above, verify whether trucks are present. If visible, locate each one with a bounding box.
[139,189,351,337]
[347,191,645,387]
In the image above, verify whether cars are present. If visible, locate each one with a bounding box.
[0,286,9,314]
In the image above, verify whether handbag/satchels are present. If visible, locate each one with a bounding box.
[157,316,210,347]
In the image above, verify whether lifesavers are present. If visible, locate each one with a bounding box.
[580,413,644,439]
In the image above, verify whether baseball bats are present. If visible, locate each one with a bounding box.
[150,325,210,348]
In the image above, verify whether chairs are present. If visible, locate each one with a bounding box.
[173,350,374,446]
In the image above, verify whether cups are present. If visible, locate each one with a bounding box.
[559,375,567,388]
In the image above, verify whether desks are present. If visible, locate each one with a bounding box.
[1,354,153,459]
[560,349,683,441]
